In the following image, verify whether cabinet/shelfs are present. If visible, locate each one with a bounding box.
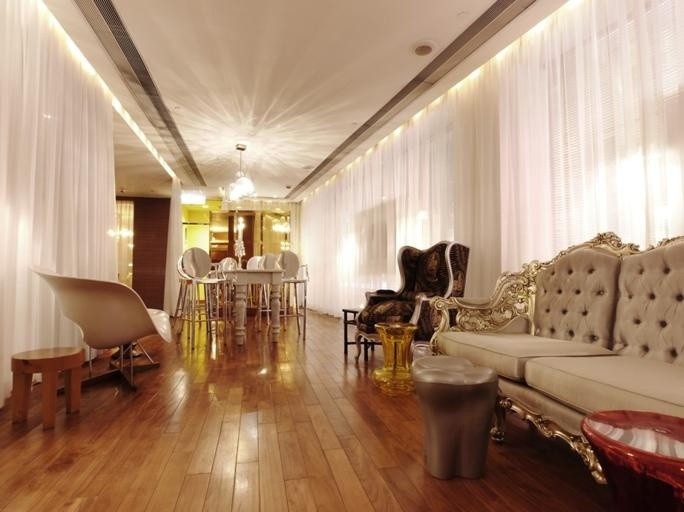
[208,210,255,270]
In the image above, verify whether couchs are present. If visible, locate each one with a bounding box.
[429,231,684,486]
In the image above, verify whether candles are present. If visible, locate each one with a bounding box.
[237,217,246,241]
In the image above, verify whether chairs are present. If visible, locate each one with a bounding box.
[34,269,172,396]
[354,240,470,362]
[172,248,310,350]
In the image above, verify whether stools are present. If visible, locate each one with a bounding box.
[410,356,498,480]
[342,307,375,362]
[11,347,85,432]
